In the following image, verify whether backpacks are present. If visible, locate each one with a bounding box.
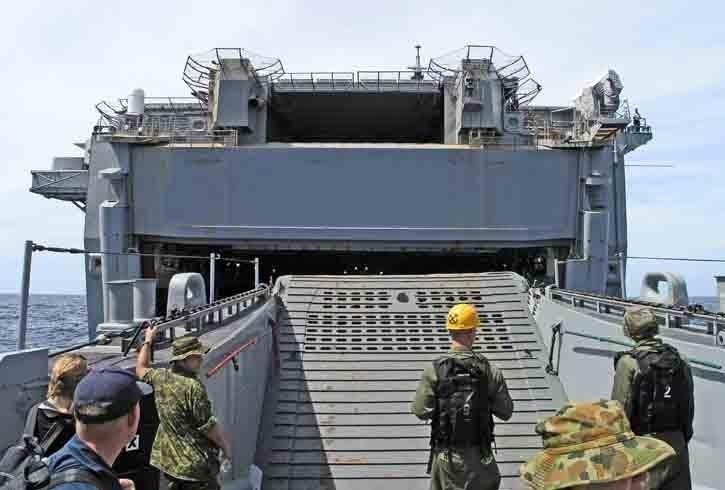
[0,403,110,490]
[614,343,687,432]
[431,356,493,446]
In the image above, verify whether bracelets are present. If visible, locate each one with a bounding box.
[142,341,150,346]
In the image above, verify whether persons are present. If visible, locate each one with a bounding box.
[23,352,89,458]
[611,304,696,490]
[135,325,233,490]
[36,365,154,490]
[409,302,515,490]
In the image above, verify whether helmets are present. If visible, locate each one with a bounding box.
[447,304,480,329]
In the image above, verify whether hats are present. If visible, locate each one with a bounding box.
[74,365,154,425]
[168,337,211,364]
[623,307,658,337]
